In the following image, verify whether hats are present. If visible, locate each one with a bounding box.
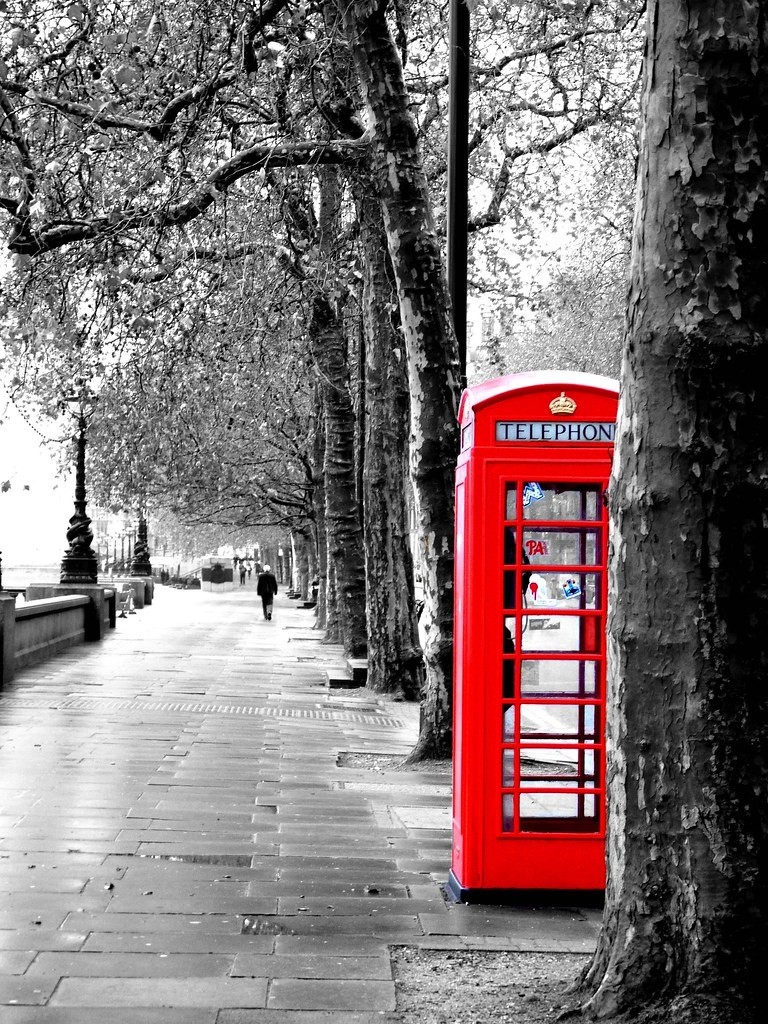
[263,564,271,572]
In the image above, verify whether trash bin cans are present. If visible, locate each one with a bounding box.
[312,580,319,600]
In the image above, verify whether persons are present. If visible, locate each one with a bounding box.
[254,561,270,575]
[161,569,200,585]
[210,561,225,583]
[257,565,278,620]
[233,554,252,584]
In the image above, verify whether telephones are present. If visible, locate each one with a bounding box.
[504,529,532,609]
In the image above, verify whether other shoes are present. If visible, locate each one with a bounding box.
[265,613,271,620]
